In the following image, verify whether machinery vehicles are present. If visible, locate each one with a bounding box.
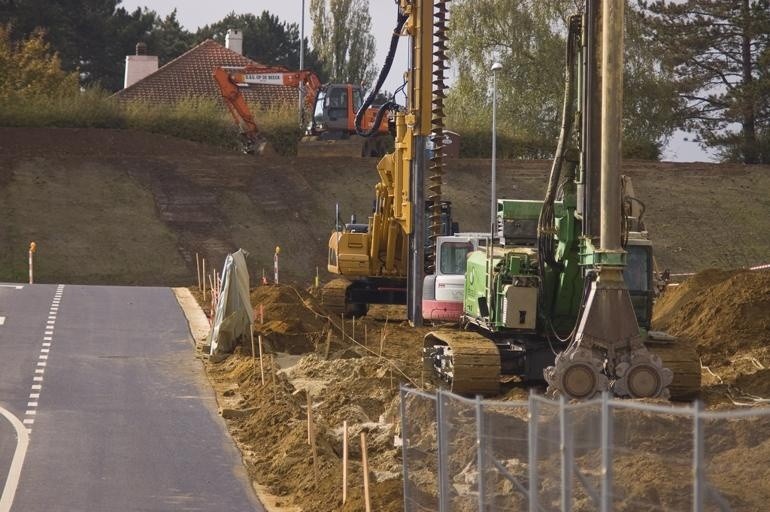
[208,65,461,163]
[423,2,705,403]
[320,1,460,317]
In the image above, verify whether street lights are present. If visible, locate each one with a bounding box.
[490,62,503,235]
